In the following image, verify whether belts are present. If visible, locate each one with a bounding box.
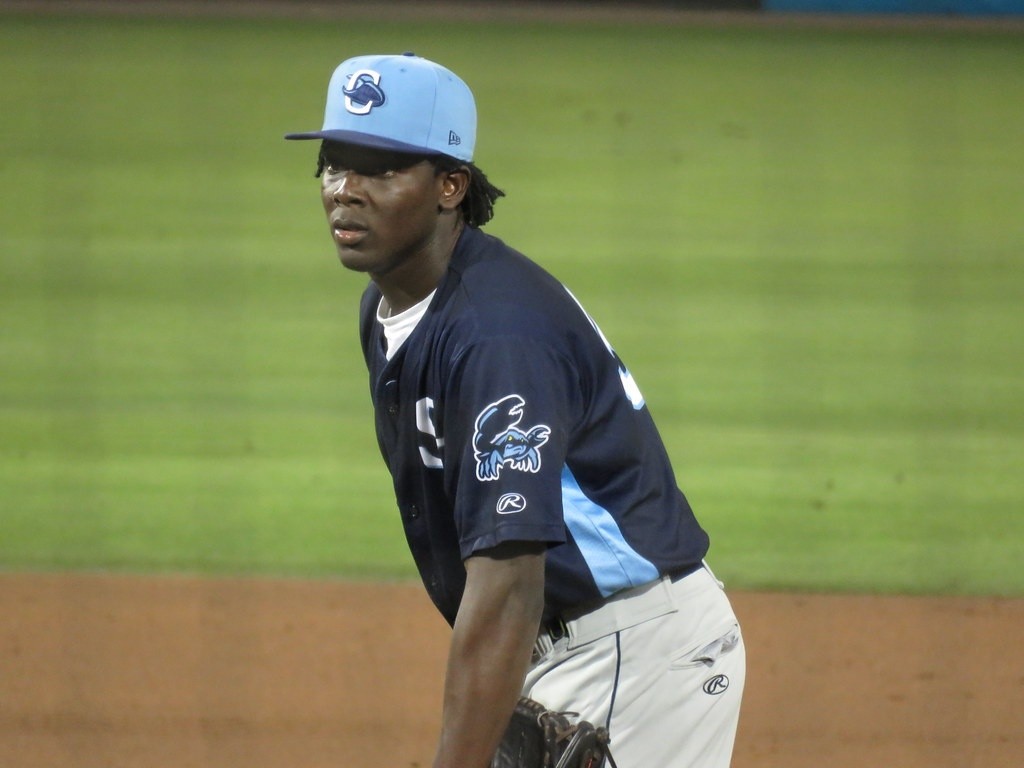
[545,555,703,642]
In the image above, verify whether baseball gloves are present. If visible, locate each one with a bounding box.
[486,691,618,768]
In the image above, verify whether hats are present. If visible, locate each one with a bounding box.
[284,51,478,165]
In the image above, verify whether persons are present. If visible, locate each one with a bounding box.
[284,52,748,768]
